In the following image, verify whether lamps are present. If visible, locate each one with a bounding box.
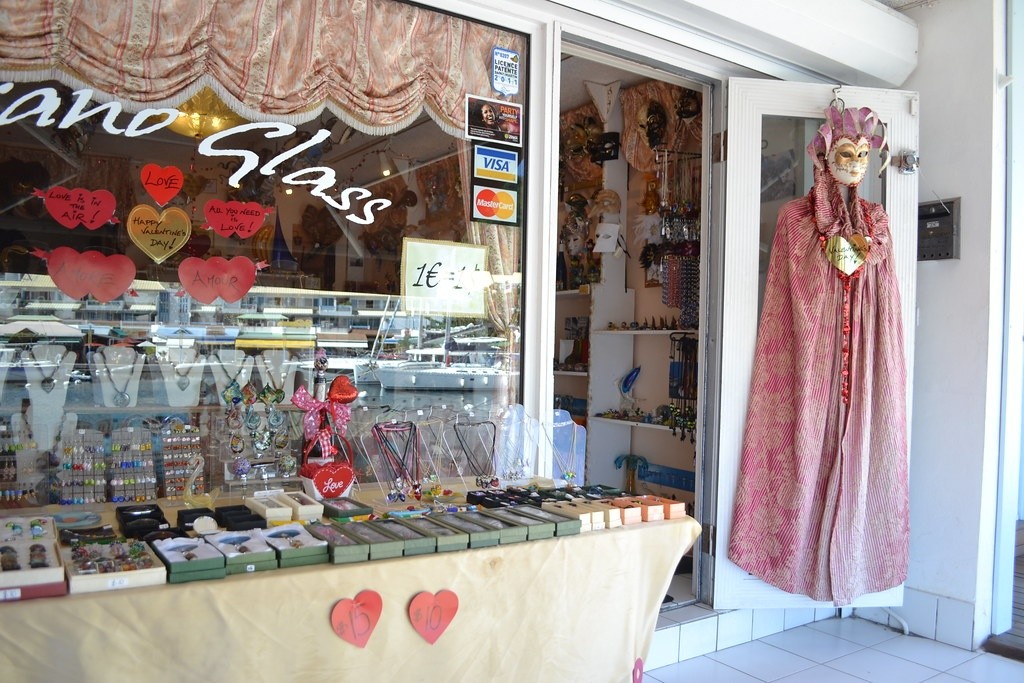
[148,85,243,151]
[378,151,392,177]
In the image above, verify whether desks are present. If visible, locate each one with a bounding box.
[0,477,702,683]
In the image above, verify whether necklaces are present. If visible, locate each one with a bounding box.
[649,147,700,446]
[0,336,299,407]
[360,406,580,502]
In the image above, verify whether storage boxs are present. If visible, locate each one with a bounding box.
[0,485,687,601]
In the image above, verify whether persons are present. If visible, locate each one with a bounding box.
[475,102,516,134]
[729,132,911,608]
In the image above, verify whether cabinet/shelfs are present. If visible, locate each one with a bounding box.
[555,56,698,576]
